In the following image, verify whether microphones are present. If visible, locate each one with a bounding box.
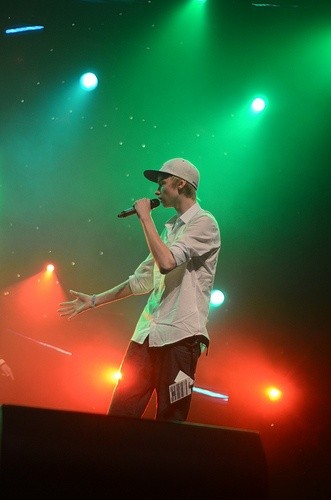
[118,199,160,217]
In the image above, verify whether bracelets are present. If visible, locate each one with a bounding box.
[90,294,96,309]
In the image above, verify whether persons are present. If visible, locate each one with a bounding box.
[57,157,222,423]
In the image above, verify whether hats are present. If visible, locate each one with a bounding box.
[143,158,200,190]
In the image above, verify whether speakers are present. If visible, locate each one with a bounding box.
[0,404,274,500]
